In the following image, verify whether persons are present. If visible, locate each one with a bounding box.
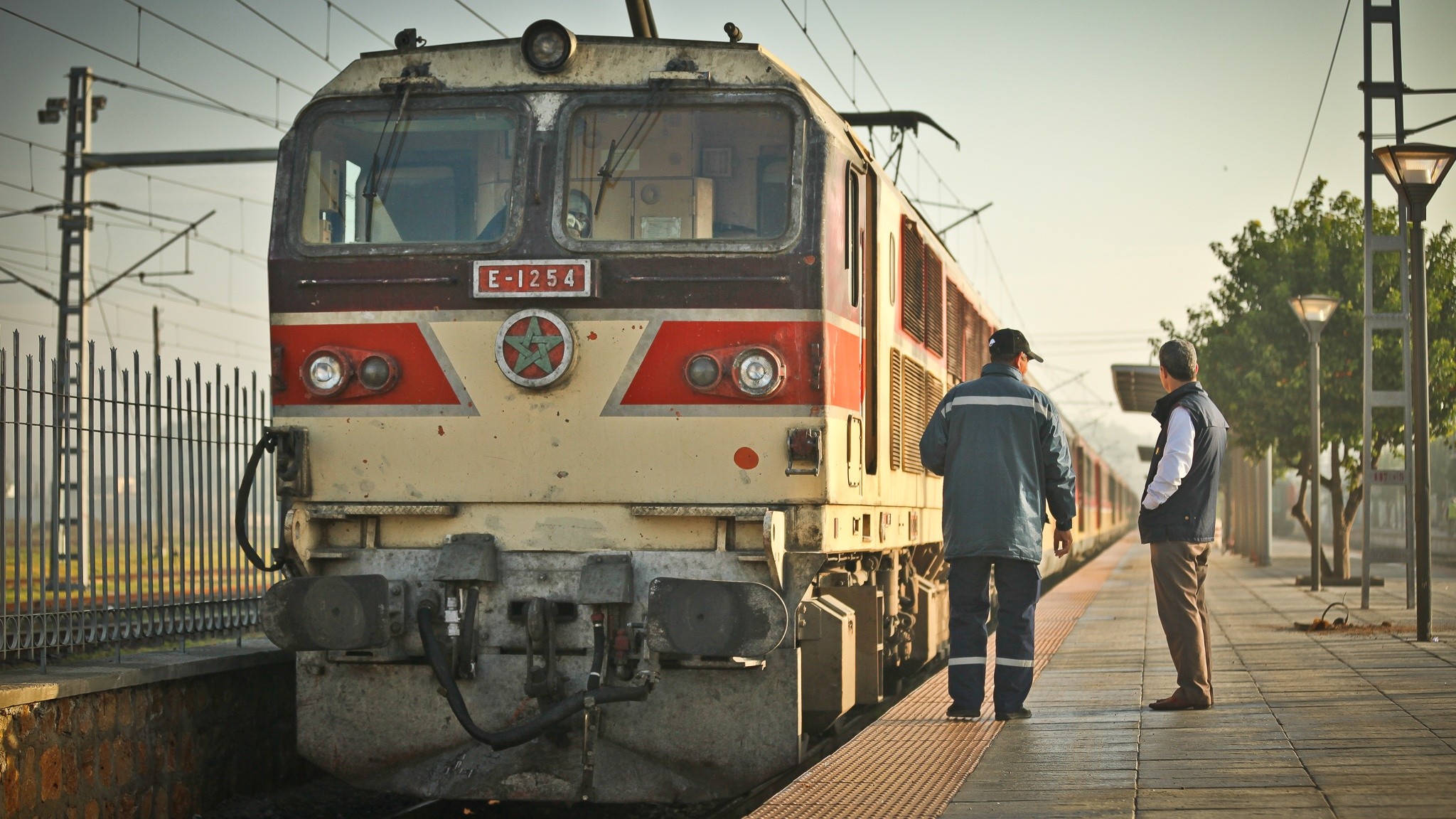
[1140,340,1228,711]
[921,329,1078,721]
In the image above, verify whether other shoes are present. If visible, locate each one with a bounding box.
[1149,693,1215,711]
[994,705,1033,721]
[946,702,981,718]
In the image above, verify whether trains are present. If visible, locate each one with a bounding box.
[232,19,1144,813]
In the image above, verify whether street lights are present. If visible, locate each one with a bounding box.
[1371,142,1456,642]
[1288,293,1343,593]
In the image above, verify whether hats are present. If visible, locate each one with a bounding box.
[989,328,1043,365]
